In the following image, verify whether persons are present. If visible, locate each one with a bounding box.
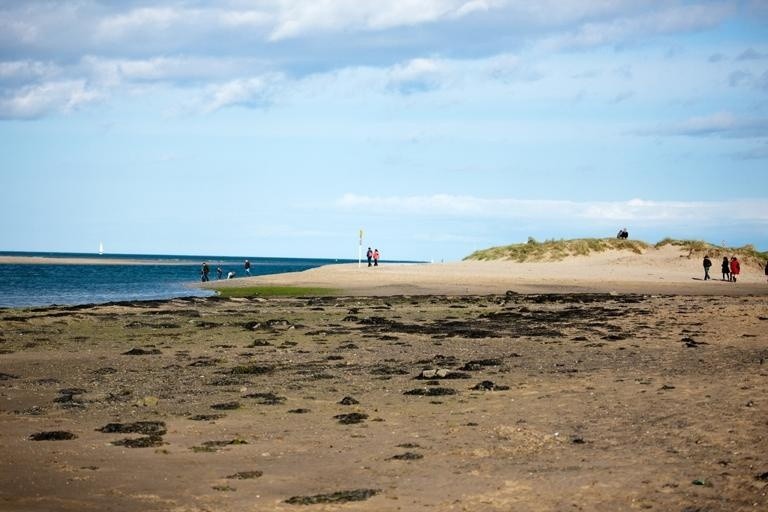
[765,261,768,281]
[199,265,237,282]
[721,257,730,281]
[617,228,628,240]
[367,247,373,267]
[245,260,251,277]
[731,257,740,282]
[729,257,734,280]
[373,249,379,266]
[703,255,712,280]
